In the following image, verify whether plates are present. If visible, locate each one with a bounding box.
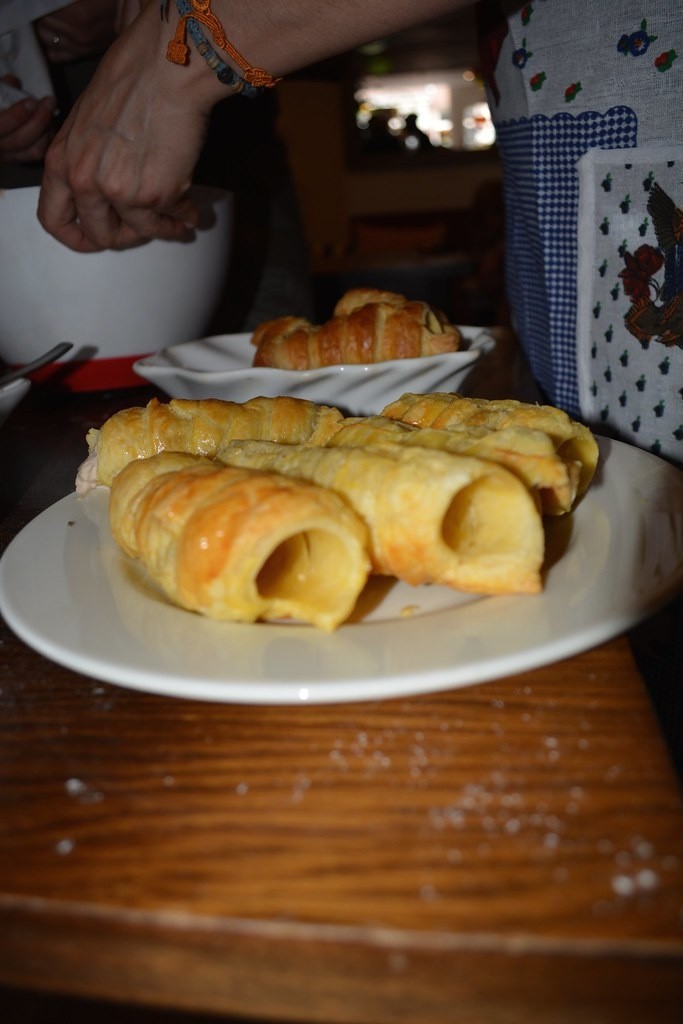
[0,434,683,705]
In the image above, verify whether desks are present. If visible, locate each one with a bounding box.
[0,353,683,1024]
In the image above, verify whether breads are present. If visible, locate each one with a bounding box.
[70,288,597,632]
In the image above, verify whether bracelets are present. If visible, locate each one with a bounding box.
[167,0,285,89]
[176,1,256,96]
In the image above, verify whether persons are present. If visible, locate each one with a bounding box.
[0,0,683,462]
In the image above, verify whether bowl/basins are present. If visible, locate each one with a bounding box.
[0,186,234,390]
[1,377,31,425]
[132,325,497,415]
